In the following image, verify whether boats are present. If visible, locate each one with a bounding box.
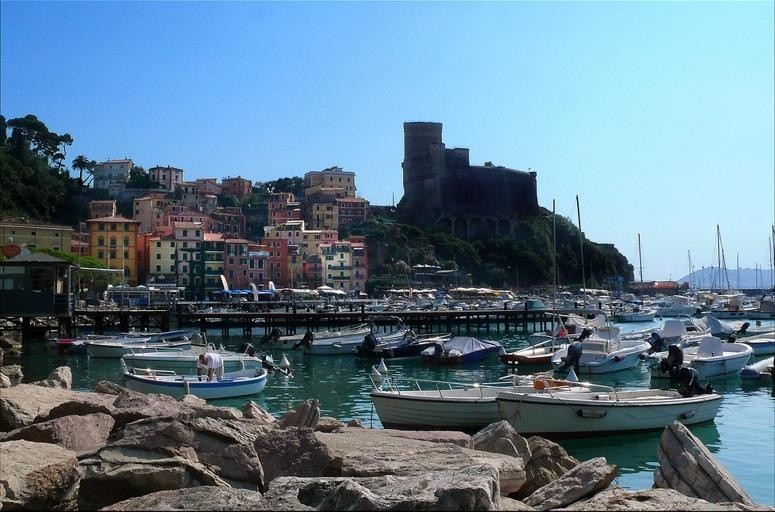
[58,328,194,349]
[562,420,724,473]
[550,310,652,372]
[497,331,582,365]
[369,333,452,356]
[351,329,416,358]
[734,330,774,355]
[642,312,738,346]
[291,327,414,356]
[494,374,726,440]
[206,391,268,412]
[367,356,594,432]
[637,335,753,380]
[271,322,380,351]
[82,338,215,361]
[740,355,774,379]
[116,351,268,401]
[419,336,503,364]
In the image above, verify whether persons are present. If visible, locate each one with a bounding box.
[196,353,225,382]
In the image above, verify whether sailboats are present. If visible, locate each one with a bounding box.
[526,197,598,346]
[563,195,610,340]
[309,222,775,323]
